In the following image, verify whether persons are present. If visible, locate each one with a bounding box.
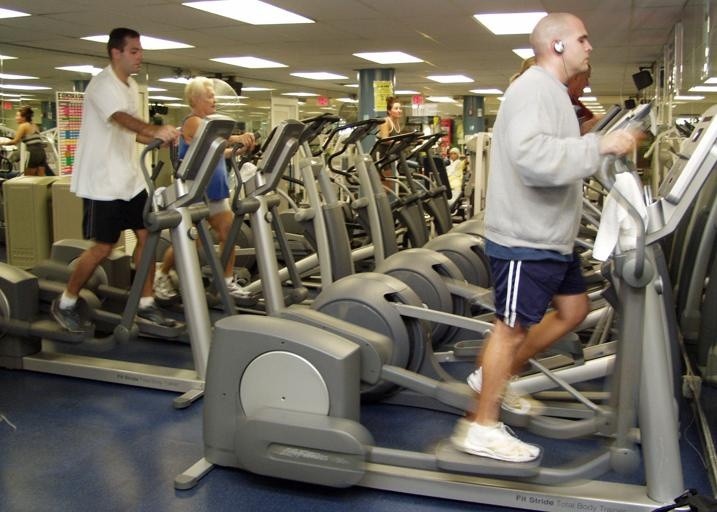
[151,76,257,303]
[50,27,183,335]
[0,106,47,177]
[444,147,465,207]
[565,64,609,137]
[370,95,402,198]
[451,12,647,463]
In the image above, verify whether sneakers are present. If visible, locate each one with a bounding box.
[152,274,177,301]
[226,275,253,300]
[136,301,177,327]
[466,366,532,416]
[462,421,540,463]
[50,297,86,333]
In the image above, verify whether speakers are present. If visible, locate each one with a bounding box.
[631,70,654,89]
[624,99,636,110]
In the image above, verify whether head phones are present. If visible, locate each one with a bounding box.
[554,40,563,53]
